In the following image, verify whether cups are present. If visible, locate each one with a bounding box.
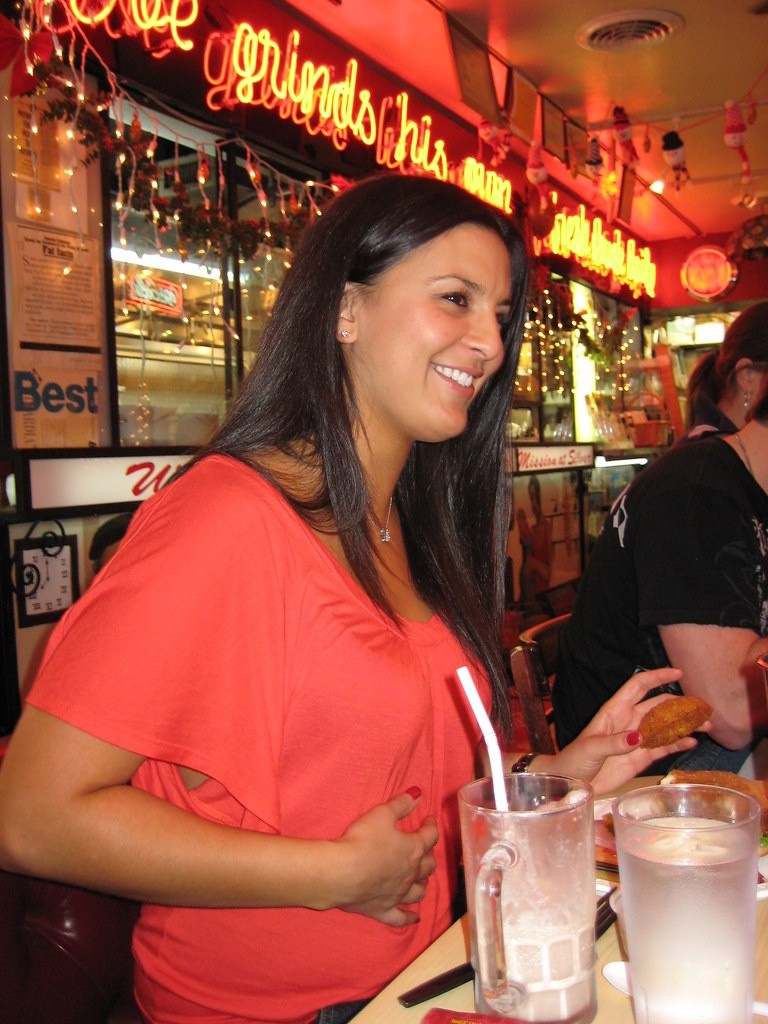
[612,783,761,1024]
[657,421,669,446]
[461,774,602,1024]
[634,421,657,448]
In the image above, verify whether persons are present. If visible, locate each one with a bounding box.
[550,301,768,785]
[0,166,710,1022]
[515,476,554,597]
[88,512,132,573]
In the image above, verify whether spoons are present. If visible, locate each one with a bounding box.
[602,961,768,1017]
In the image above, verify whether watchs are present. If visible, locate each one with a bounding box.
[511,752,537,774]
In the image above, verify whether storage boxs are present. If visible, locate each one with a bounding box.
[630,421,670,448]
[345,776,768,1024]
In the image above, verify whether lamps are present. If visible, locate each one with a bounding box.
[649,165,669,194]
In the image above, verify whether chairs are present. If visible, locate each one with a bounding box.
[1,867,146,1023]
[510,614,573,755]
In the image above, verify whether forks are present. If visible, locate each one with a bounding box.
[399,885,620,1006]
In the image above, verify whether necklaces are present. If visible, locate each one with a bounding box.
[734,434,753,475]
[366,495,394,543]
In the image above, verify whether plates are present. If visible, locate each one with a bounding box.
[589,792,667,853]
